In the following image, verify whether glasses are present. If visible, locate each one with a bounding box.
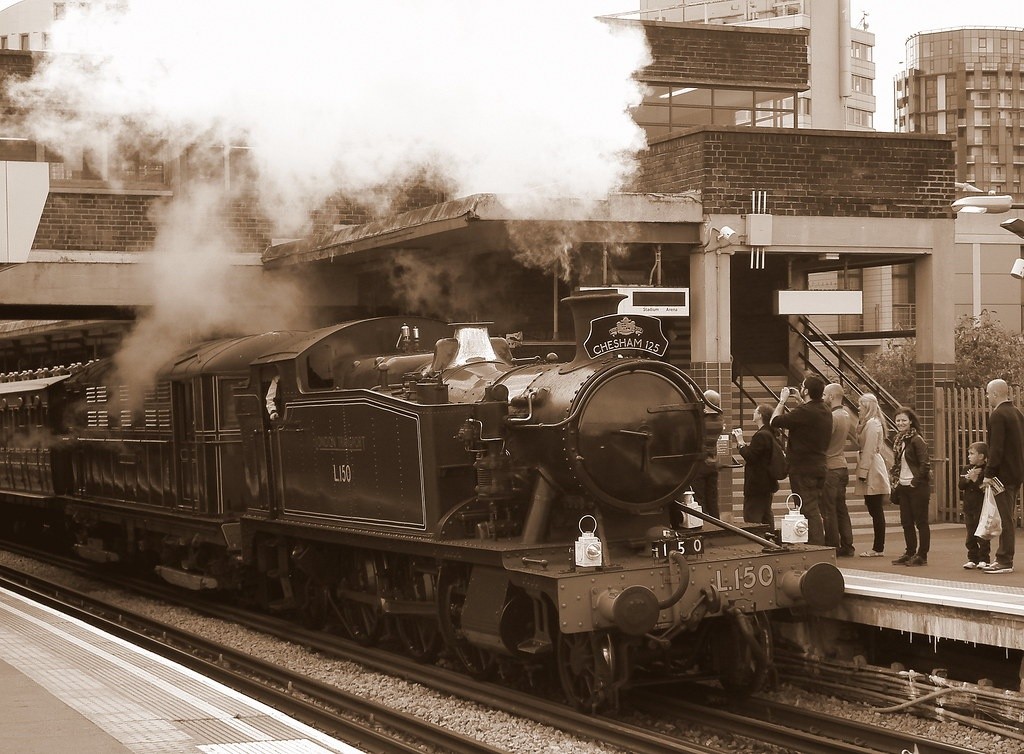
[799,383,806,389]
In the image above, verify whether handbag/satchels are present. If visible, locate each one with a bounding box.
[973,486,1002,541]
[889,483,900,505]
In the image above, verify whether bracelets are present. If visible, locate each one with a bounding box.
[779,401,785,405]
[800,399,804,403]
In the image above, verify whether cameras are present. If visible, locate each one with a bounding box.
[789,389,796,394]
[731,428,742,435]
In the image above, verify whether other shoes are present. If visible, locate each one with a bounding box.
[905,554,927,566]
[845,545,856,556]
[836,548,850,559]
[976,561,987,569]
[891,553,911,565]
[859,549,883,557]
[963,561,976,569]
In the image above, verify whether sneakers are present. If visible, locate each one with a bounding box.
[990,557,999,565]
[984,564,1014,574]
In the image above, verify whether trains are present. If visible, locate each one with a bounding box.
[0,285,846,717]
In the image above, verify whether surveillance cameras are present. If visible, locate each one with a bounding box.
[720,226,738,243]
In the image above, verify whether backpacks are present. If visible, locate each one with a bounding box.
[764,429,790,480]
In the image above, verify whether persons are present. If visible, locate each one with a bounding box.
[769,373,843,557]
[889,407,931,567]
[735,403,780,530]
[818,383,856,558]
[958,441,991,569]
[266,376,286,421]
[854,393,892,558]
[983,379,1024,574]
